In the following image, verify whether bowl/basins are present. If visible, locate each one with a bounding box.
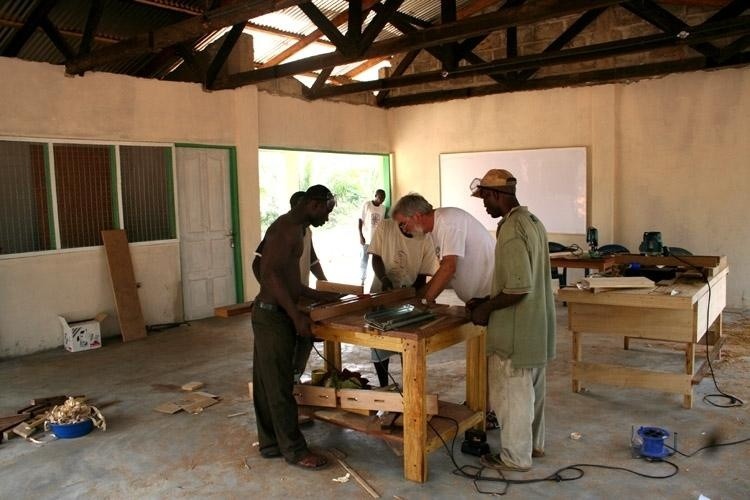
[47,418,95,439]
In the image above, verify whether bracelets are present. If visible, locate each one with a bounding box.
[380,276,387,280]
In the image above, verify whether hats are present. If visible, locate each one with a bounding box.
[470,169,516,197]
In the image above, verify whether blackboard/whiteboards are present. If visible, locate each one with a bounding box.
[439,145,587,236]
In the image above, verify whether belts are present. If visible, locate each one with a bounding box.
[254,296,284,312]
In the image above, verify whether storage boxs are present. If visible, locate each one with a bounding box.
[58,311,109,353]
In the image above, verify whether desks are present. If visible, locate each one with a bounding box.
[549,251,630,307]
[555,265,729,408]
[292,293,488,482]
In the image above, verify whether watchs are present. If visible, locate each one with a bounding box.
[419,296,434,306]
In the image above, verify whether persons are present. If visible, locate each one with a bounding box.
[393,194,499,430]
[367,220,442,388]
[358,189,390,286]
[252,191,329,426]
[252,184,335,471]
[465,170,558,472]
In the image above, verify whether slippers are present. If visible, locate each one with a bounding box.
[480,454,528,472]
[295,453,328,470]
